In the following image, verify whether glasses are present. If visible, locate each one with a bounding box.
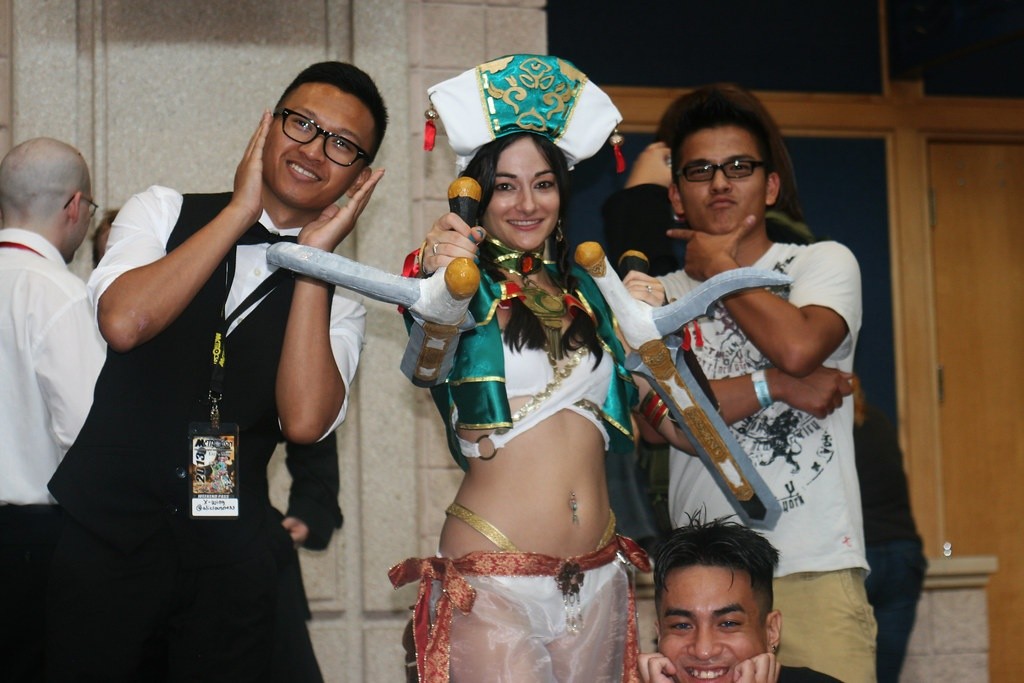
[273,108,371,167]
[64,195,98,217]
[676,160,772,182]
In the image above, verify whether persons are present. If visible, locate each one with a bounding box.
[1,61,390,683]
[396,54,666,683]
[606,84,931,683]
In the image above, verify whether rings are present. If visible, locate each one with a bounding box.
[432,244,439,256]
[646,286,652,293]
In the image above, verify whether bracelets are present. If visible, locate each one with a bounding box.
[750,369,774,408]
